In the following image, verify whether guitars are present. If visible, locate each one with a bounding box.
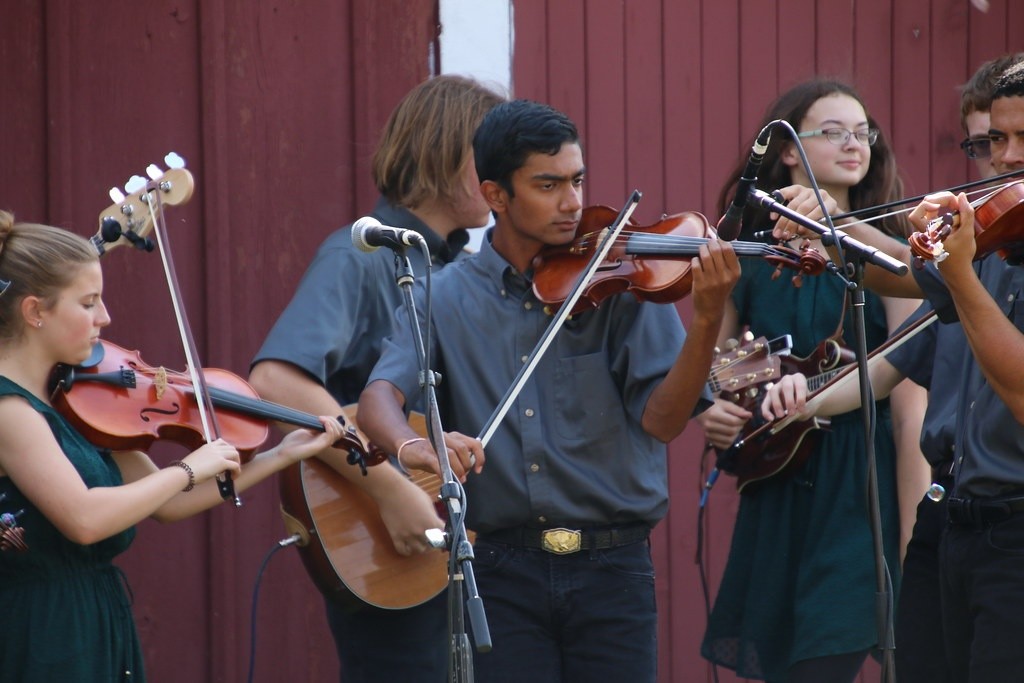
[712,338,858,495]
[277,330,794,610]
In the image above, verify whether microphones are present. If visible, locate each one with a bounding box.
[717,125,774,242]
[351,216,425,253]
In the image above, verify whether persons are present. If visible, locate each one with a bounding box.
[699,57,1024,683]
[251,75,740,683]
[0,208,345,683]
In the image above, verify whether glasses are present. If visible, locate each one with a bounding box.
[960,138,992,159]
[797,128,880,146]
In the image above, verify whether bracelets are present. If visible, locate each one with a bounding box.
[169,460,195,492]
[398,438,425,477]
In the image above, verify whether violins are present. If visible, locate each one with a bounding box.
[531,203,828,319]
[46,339,389,478]
[907,179,1024,269]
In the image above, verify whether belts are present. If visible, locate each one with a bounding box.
[477,523,650,555]
[934,462,956,476]
[947,496,1024,525]
